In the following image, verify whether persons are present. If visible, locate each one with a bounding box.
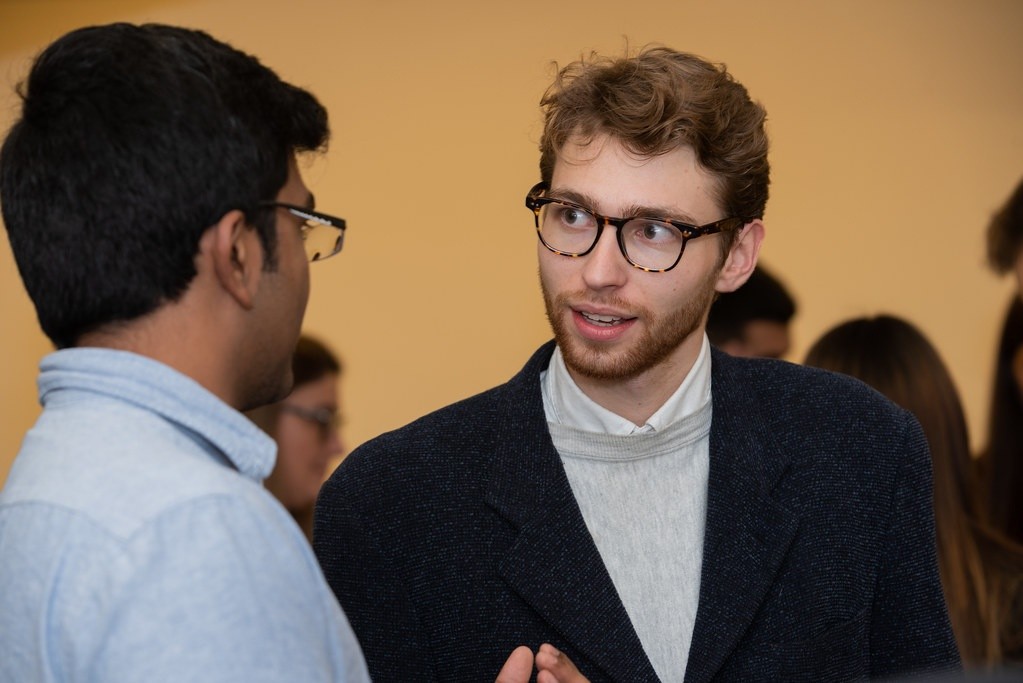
[312,31,965,682]
[987,185,1023,293]
[0,23,592,683]
[977,296,1023,553]
[806,311,1023,683]
[242,333,341,536]
[705,266,795,367]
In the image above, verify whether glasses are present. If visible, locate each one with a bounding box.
[246,199,346,263]
[279,402,337,431]
[525,182,749,274]
